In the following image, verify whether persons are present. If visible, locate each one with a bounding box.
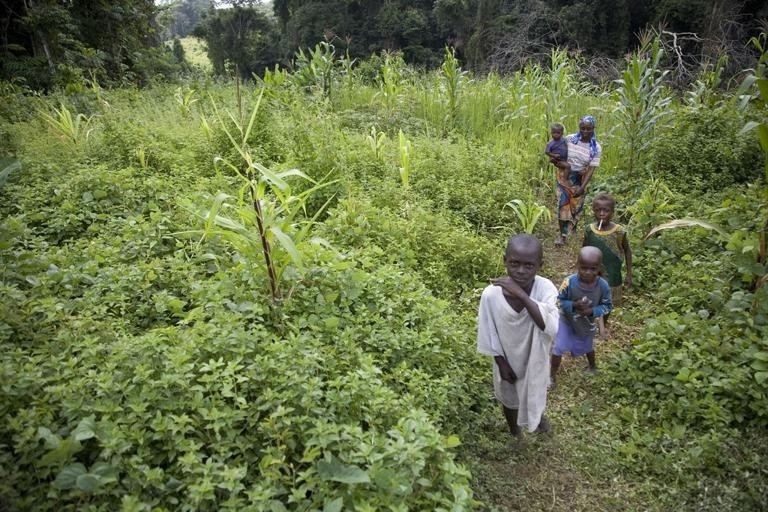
[545,123,571,182]
[582,194,635,339]
[476,232,560,438]
[547,115,601,248]
[550,246,612,376]
